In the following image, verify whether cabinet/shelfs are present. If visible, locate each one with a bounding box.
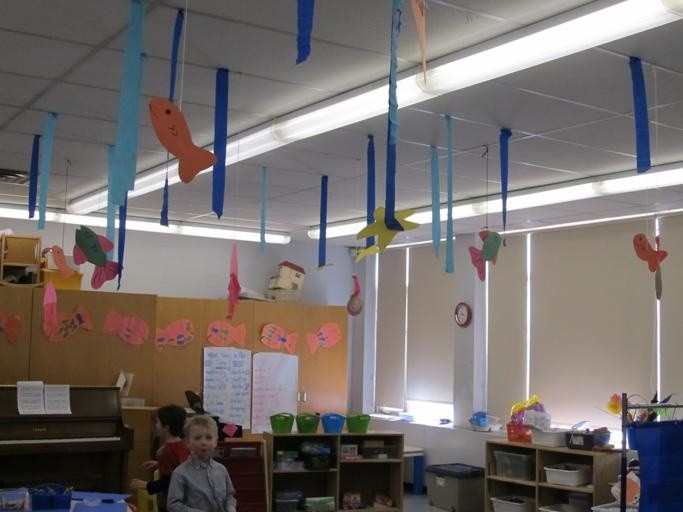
[213,431,268,511]
[121,406,159,486]
[484,439,623,512]
[263,433,404,511]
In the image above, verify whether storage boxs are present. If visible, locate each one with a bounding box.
[493,450,535,481]
[544,462,593,487]
[424,462,484,511]
[532,428,569,447]
[567,432,610,450]
[490,493,533,512]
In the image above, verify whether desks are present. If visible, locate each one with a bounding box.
[403,444,424,495]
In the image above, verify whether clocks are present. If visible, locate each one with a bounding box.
[453,303,472,327]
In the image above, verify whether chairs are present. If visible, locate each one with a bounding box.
[137,469,160,512]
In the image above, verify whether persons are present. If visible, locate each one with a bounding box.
[129,404,191,512]
[166,414,237,512]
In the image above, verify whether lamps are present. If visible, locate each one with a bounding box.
[0,206,292,245]
[306,162,683,241]
[61,1,682,216]
